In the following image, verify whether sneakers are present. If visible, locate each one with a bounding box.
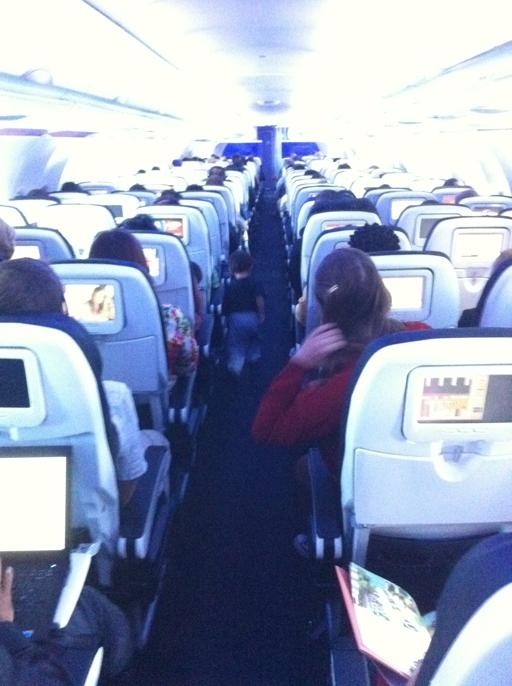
[291,532,313,560]
[230,356,262,380]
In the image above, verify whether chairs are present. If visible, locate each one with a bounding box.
[276,153,510,686]
[0,154,265,686]
